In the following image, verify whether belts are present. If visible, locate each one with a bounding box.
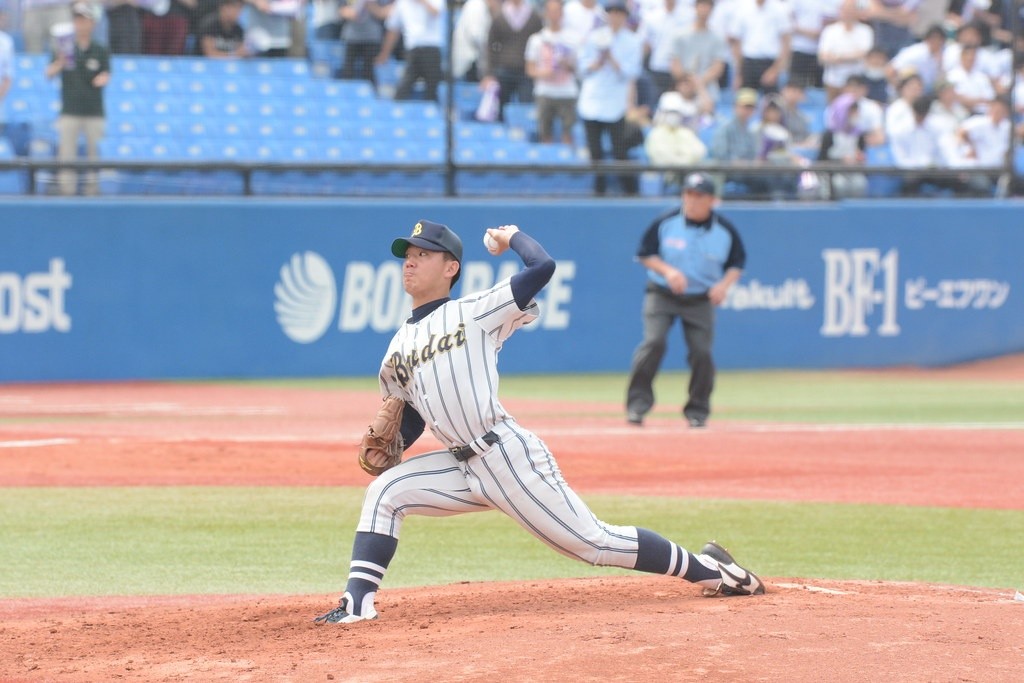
[449,431,499,462]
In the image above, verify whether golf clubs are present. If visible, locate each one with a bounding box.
[357,395,407,477]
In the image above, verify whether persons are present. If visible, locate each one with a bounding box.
[0,30,13,98]
[313,221,766,623]
[625,174,747,427]
[106,0,1024,200]
[47,6,112,194]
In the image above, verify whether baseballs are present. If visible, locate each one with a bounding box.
[484,228,498,250]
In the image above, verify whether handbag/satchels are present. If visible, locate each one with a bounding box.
[476,80,500,121]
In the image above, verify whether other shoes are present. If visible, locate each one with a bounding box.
[627,413,642,424]
[688,418,705,428]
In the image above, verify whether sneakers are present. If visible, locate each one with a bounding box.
[700,540,765,596]
[310,595,380,624]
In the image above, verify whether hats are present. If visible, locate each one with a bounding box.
[658,91,700,117]
[605,0,629,15]
[737,87,758,106]
[391,219,463,268]
[72,2,98,20]
[683,172,715,193]
[826,91,855,135]
